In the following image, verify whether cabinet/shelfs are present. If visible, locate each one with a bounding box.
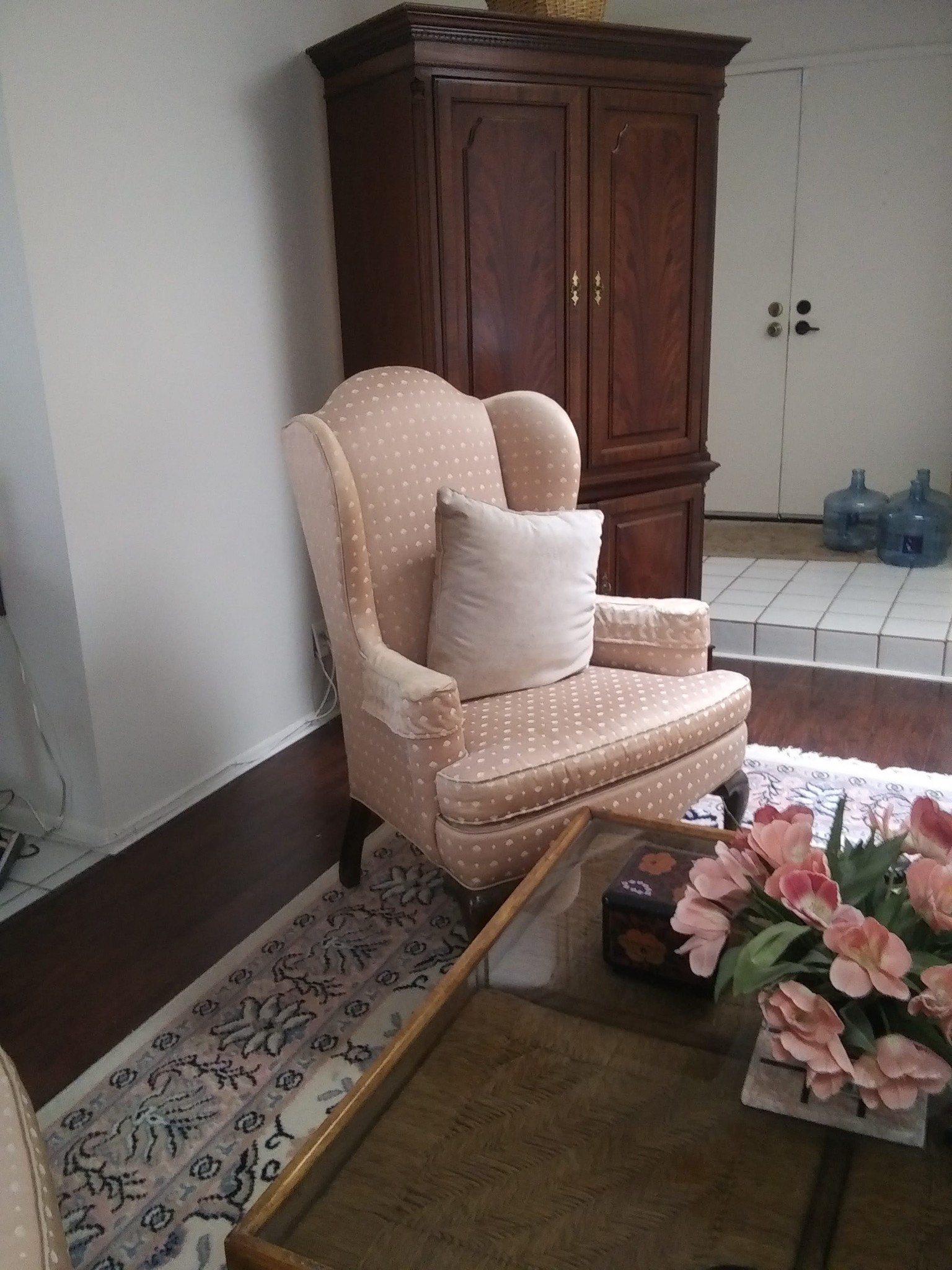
[303,1,751,669]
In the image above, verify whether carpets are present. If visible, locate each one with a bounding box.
[34,744,952,1270]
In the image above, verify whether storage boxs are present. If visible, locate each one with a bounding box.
[601,845,729,993]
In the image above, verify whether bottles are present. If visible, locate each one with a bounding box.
[887,468,952,557]
[877,479,949,567]
[822,470,890,552]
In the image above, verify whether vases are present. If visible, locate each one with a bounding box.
[739,1010,927,1151]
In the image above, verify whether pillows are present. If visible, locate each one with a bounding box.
[429,490,604,699]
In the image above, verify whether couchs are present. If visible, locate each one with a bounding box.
[280,364,754,932]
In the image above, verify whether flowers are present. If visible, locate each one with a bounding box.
[672,796,952,1111]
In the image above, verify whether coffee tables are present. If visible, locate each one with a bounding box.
[221,810,952,1270]
